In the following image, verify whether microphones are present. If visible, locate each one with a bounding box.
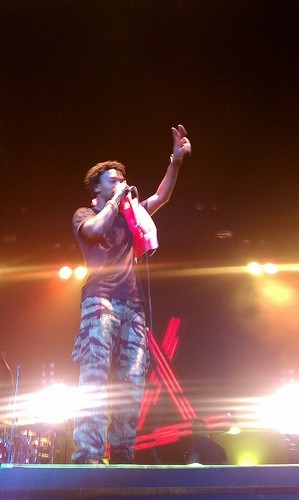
[125,186,135,192]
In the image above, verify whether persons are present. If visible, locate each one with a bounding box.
[184,421,227,465]
[146,416,169,466]
[72,124,191,464]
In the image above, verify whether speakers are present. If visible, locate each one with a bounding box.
[131,429,228,465]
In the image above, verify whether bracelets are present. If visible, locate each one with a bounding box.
[105,200,119,215]
[170,153,183,168]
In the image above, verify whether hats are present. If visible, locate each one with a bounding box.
[191,417,212,435]
